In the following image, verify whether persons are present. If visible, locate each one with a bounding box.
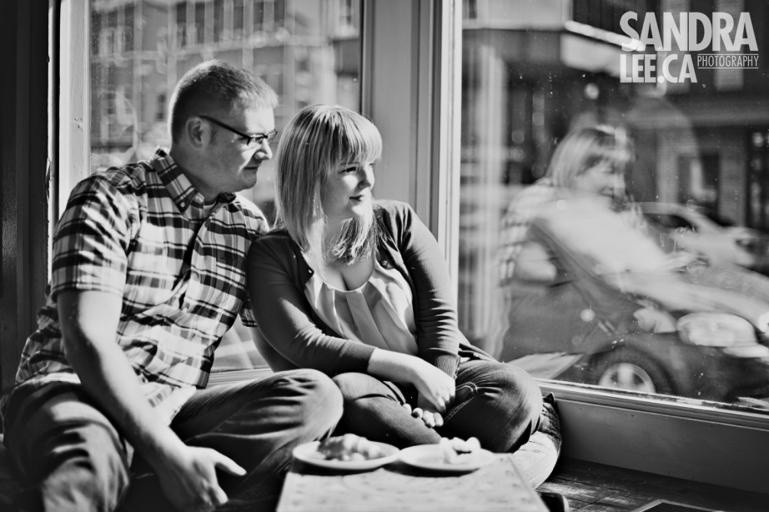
[499,123,675,386]
[241,101,545,457]
[0,60,346,512]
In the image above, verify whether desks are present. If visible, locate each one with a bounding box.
[274,452,548,512]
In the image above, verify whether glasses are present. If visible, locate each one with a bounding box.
[199,114,279,149]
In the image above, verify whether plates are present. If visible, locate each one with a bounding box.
[293,439,496,472]
